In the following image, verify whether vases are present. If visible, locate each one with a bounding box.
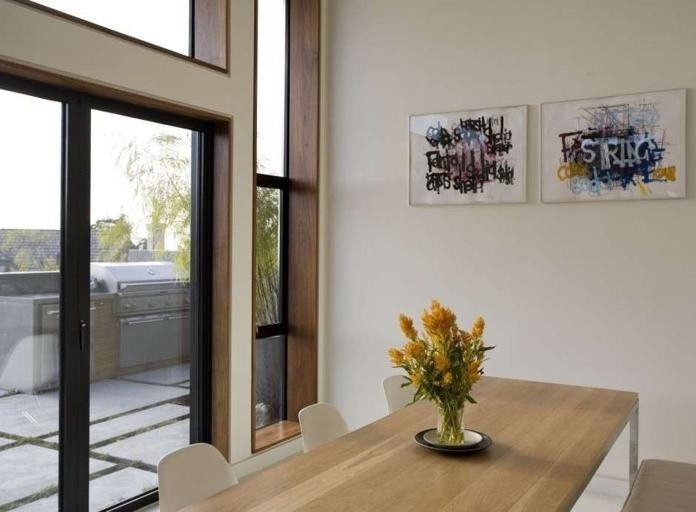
[433,404,467,446]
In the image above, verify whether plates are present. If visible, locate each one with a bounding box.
[412,427,493,455]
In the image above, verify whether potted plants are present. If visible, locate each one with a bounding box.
[111,130,283,412]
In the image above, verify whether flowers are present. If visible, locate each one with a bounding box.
[387,300,497,406]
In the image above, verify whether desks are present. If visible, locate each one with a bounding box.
[174,373,641,512]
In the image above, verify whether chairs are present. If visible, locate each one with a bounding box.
[619,457,696,512]
[297,402,350,454]
[157,442,239,512]
[381,373,419,415]
